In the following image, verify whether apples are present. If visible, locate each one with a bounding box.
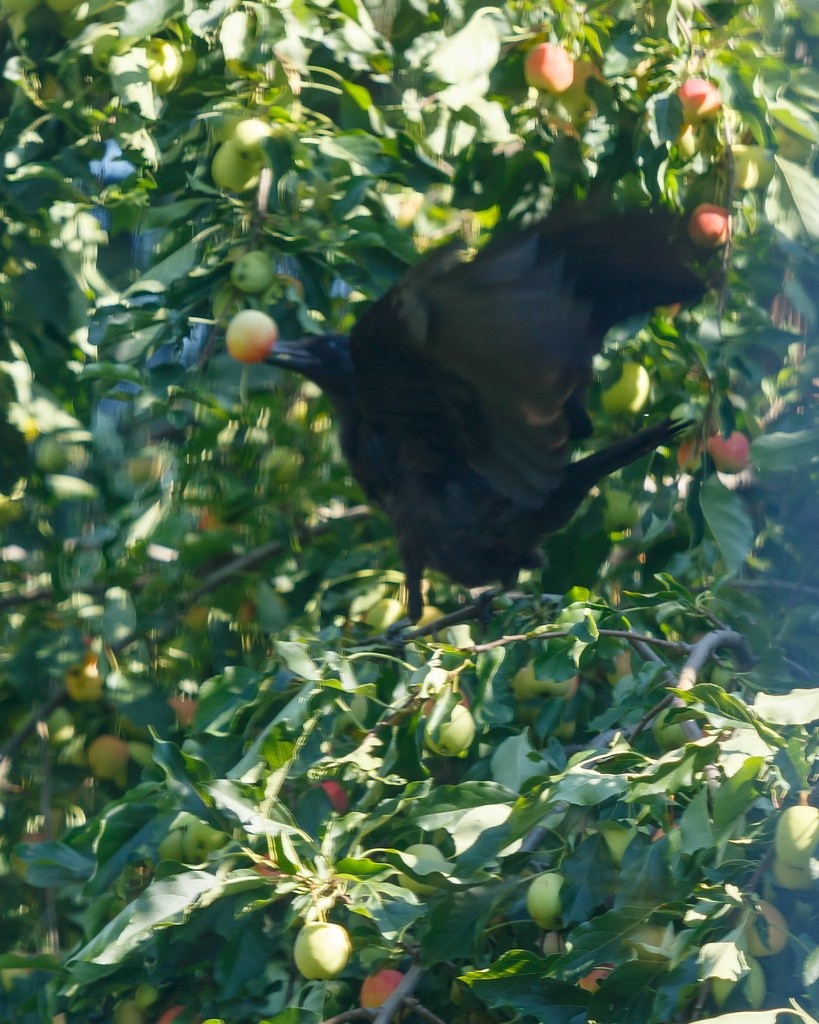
[39,41,819,1024]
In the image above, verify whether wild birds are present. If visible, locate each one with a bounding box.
[264,213,725,643]
